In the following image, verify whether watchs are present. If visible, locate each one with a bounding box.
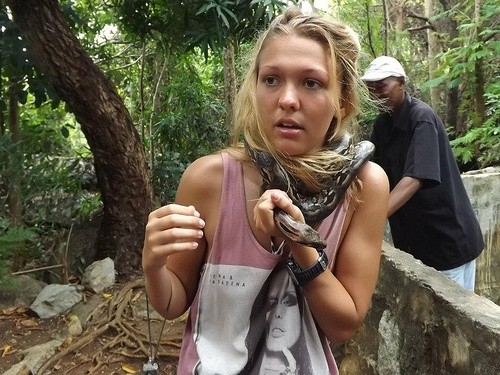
[287,249,329,287]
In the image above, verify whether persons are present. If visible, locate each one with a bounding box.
[360,56,486,292]
[238,260,315,375]
[143,5,390,375]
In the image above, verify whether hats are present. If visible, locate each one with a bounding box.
[361,56,404,81]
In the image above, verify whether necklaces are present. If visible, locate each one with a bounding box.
[270,236,288,256]
